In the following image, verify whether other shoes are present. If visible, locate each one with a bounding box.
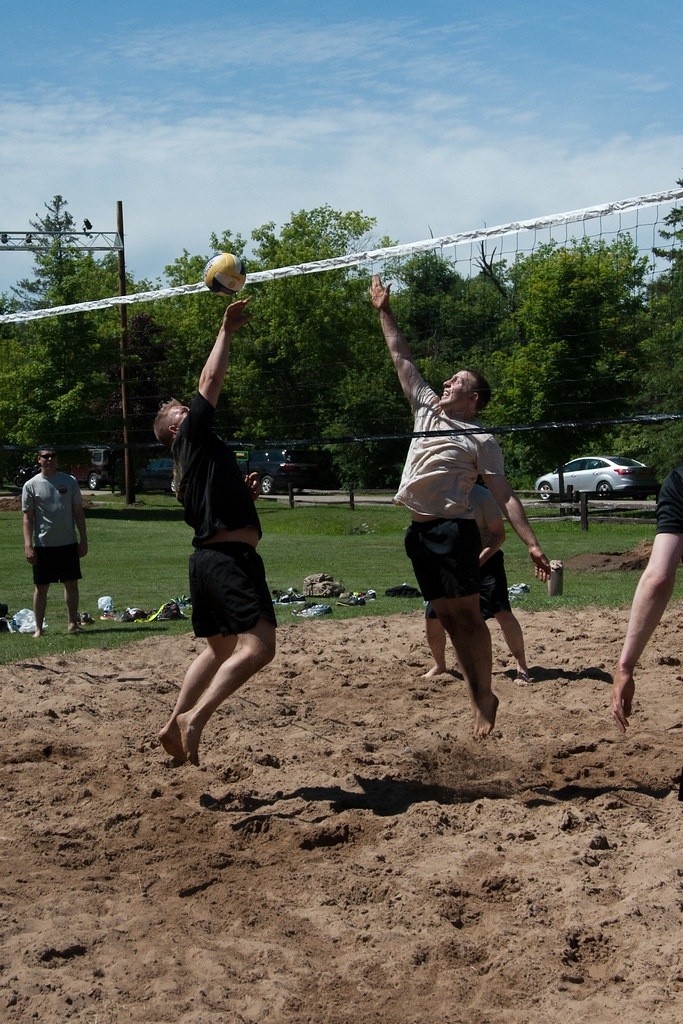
[76,613,81,624]
[291,603,332,619]
[337,597,366,607]
[171,595,192,609]
[81,613,94,625]
[366,590,376,598]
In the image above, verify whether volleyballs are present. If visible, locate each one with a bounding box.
[202,252,247,298]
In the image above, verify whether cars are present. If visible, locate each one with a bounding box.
[534,456,658,504]
[134,457,176,494]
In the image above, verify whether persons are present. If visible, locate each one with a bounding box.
[420,475,530,687]
[611,467,683,734]
[369,274,552,739]
[22,443,88,638]
[154,298,278,767]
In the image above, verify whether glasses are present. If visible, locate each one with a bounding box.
[41,453,56,459]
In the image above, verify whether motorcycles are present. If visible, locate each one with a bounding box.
[14,462,42,488]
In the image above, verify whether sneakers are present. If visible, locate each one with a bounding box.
[275,593,306,605]
[507,582,531,593]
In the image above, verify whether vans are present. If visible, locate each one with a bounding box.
[55,444,124,491]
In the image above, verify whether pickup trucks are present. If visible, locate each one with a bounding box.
[233,448,314,496]
